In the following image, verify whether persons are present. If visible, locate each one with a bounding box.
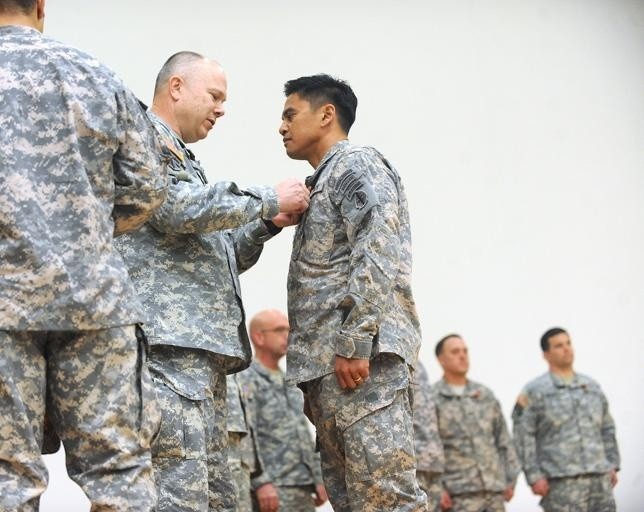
[112,52,312,511]
[279,72,427,511]
[512,328,621,511]
[226,309,329,511]
[414,334,523,511]
